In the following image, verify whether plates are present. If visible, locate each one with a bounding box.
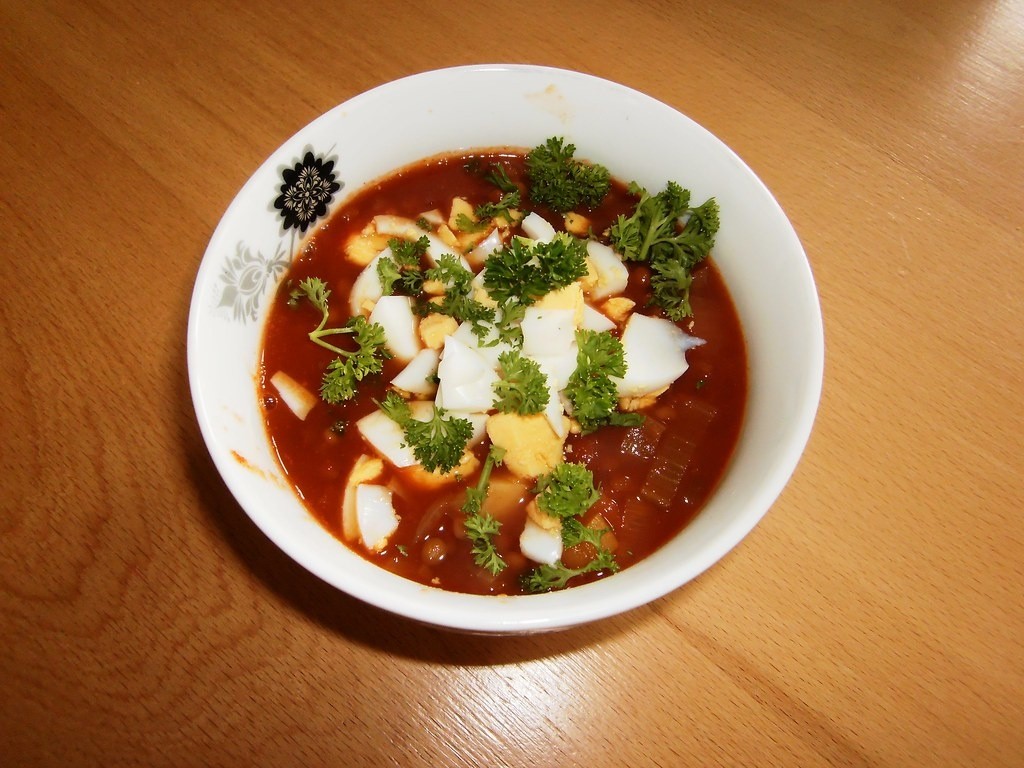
[185,65,825,637]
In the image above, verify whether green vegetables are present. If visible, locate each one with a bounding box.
[287,135,720,594]
